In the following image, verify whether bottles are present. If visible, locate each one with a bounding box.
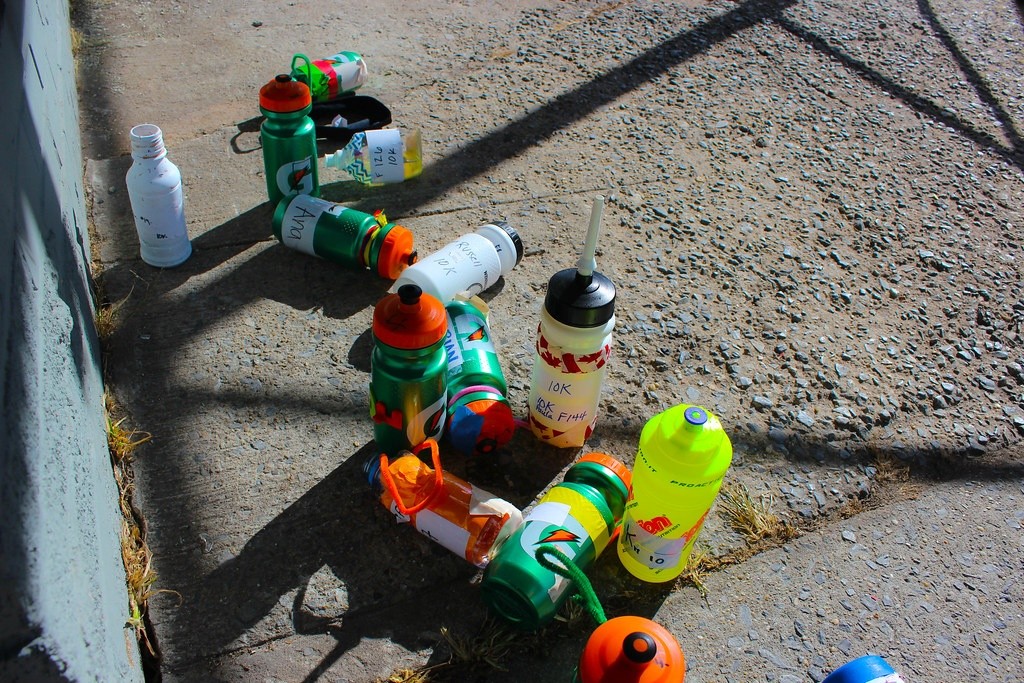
[125,124,192,268]
[820,654,904,683]
[255,50,732,683]
